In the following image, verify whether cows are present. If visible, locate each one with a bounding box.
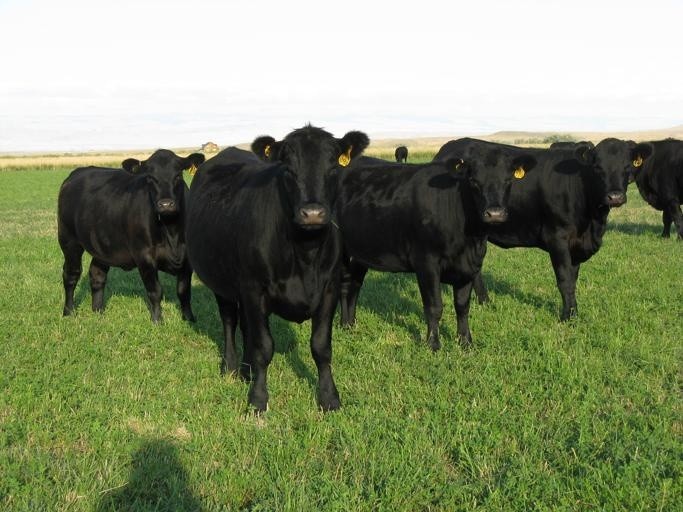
[549,141,595,150]
[56,149,206,326]
[333,145,538,355]
[394,146,408,164]
[431,137,654,325]
[183,121,370,419]
[625,137,683,243]
